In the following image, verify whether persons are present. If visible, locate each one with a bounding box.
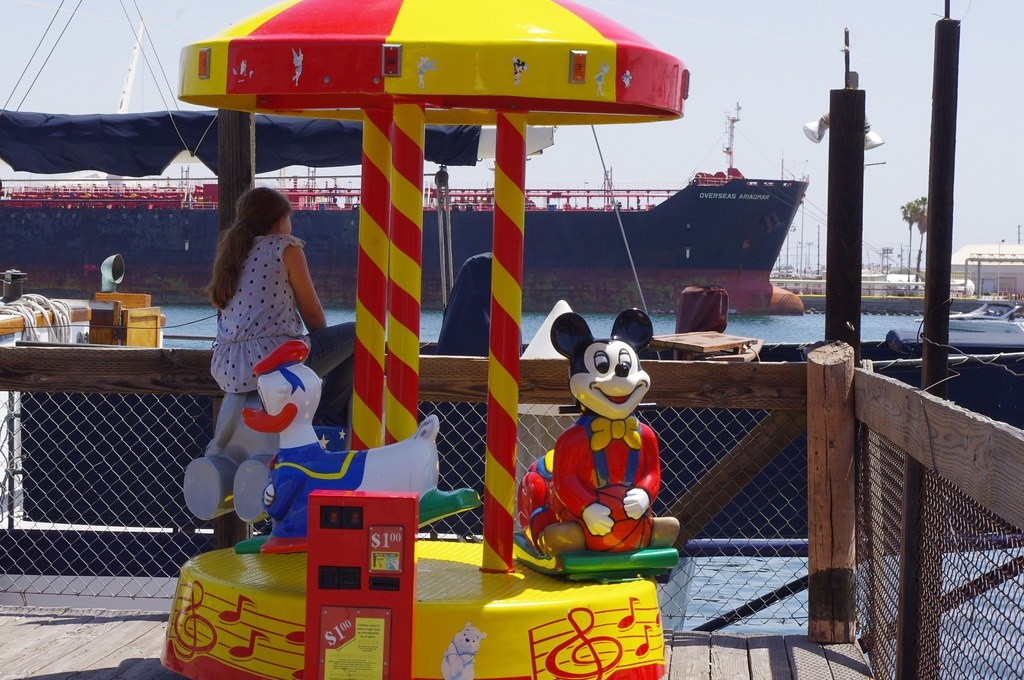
[985,310,1015,323]
[203,187,356,427]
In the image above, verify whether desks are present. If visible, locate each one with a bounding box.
[651,330,762,362]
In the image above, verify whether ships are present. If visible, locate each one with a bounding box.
[0,19,812,314]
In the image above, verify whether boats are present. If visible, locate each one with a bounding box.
[913,298,1024,334]
